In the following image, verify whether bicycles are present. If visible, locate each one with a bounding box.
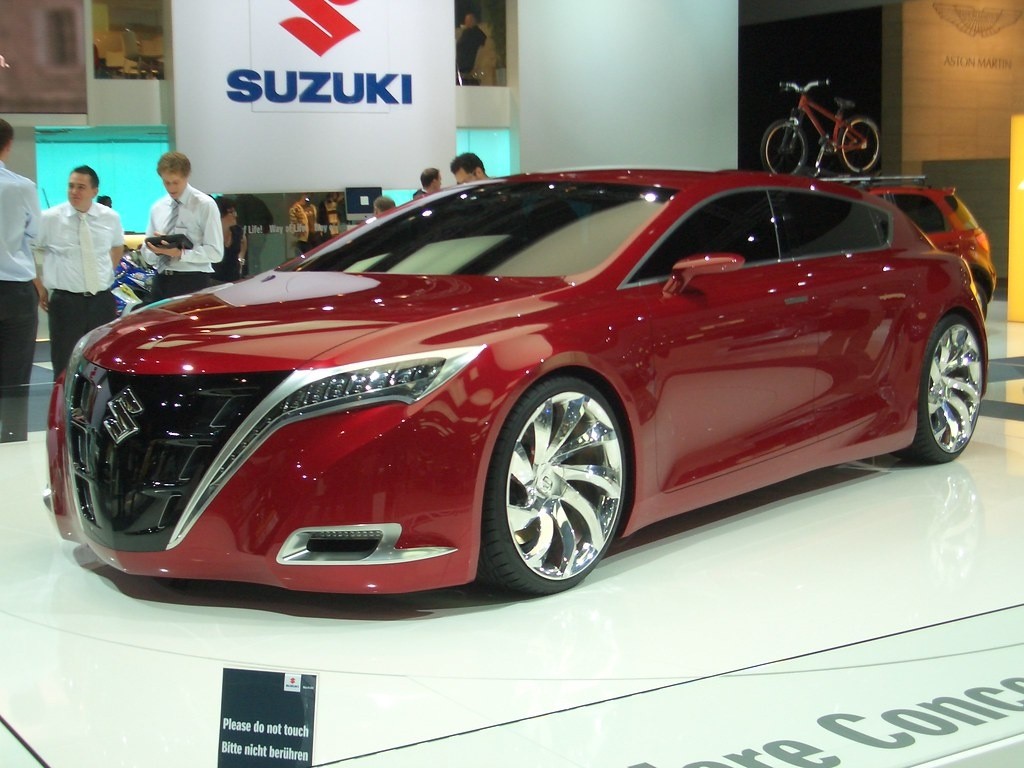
[758,78,880,176]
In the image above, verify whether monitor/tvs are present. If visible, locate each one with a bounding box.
[345,187,382,220]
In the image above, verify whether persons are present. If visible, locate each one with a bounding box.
[412,168,442,198]
[98,195,112,208]
[30,165,125,381]
[211,196,249,283]
[238,194,274,279]
[0,118,41,442]
[140,153,224,303]
[289,191,352,254]
[373,197,396,217]
[450,153,489,185]
[455,12,478,73]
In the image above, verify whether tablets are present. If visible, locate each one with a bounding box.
[145,234,193,256]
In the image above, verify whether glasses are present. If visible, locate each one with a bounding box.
[228,210,237,217]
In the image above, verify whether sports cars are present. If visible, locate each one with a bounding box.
[40,165,991,599]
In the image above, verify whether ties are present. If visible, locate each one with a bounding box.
[77,213,99,296]
[157,199,183,273]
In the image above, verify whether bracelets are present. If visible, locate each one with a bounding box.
[238,258,245,265]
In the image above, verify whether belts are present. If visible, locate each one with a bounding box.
[161,270,200,276]
[54,289,109,298]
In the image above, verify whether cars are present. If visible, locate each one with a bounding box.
[815,173,997,321]
